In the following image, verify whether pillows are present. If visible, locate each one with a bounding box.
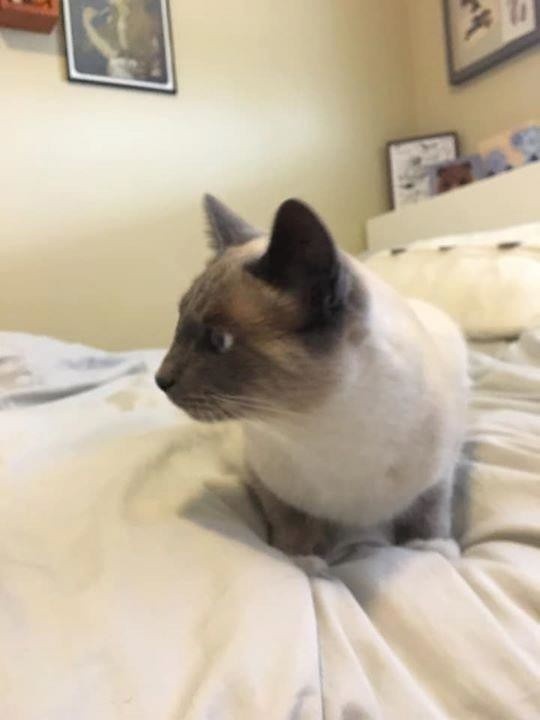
[351,216,540,341]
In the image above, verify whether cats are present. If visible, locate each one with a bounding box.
[154,194,476,576]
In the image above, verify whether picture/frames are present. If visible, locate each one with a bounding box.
[385,131,466,211]
[440,0,539,89]
[58,0,178,97]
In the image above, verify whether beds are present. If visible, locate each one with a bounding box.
[1,157,539,719]
